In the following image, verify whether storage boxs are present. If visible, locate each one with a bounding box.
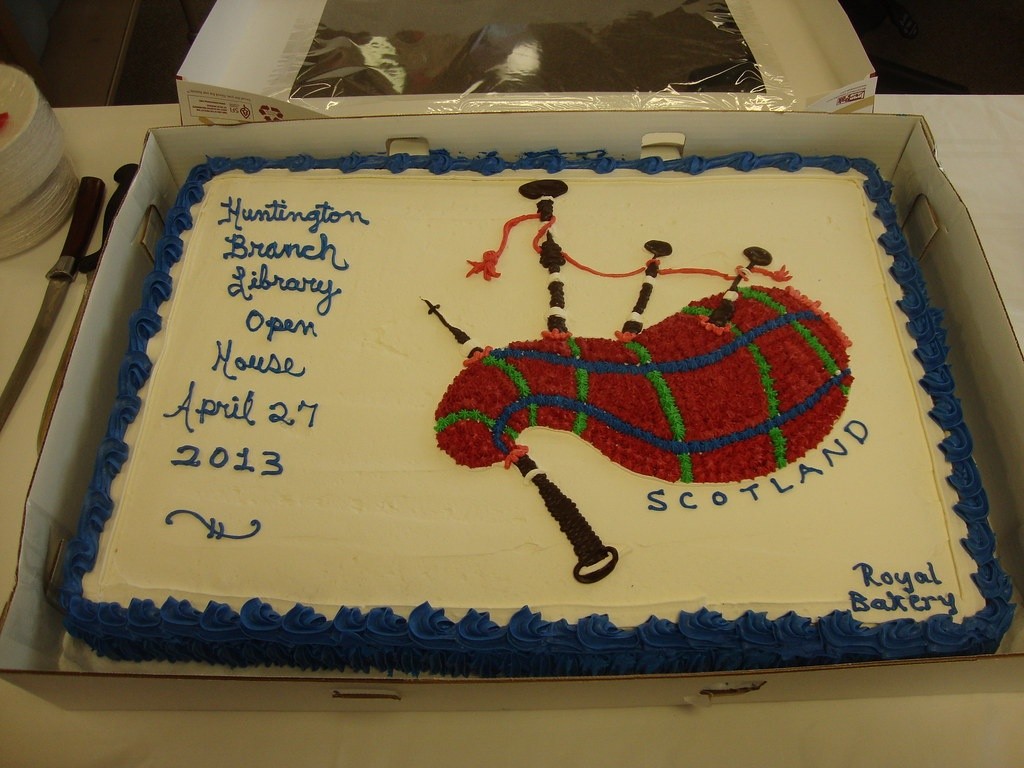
[0,0,1024,711]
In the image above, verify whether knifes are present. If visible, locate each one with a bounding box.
[0,176,105,433]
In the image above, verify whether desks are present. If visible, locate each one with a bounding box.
[0,96,1024,768]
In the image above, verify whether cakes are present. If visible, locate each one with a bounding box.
[49,154,1016,675]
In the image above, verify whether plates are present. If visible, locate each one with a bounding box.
[0,60,80,260]
